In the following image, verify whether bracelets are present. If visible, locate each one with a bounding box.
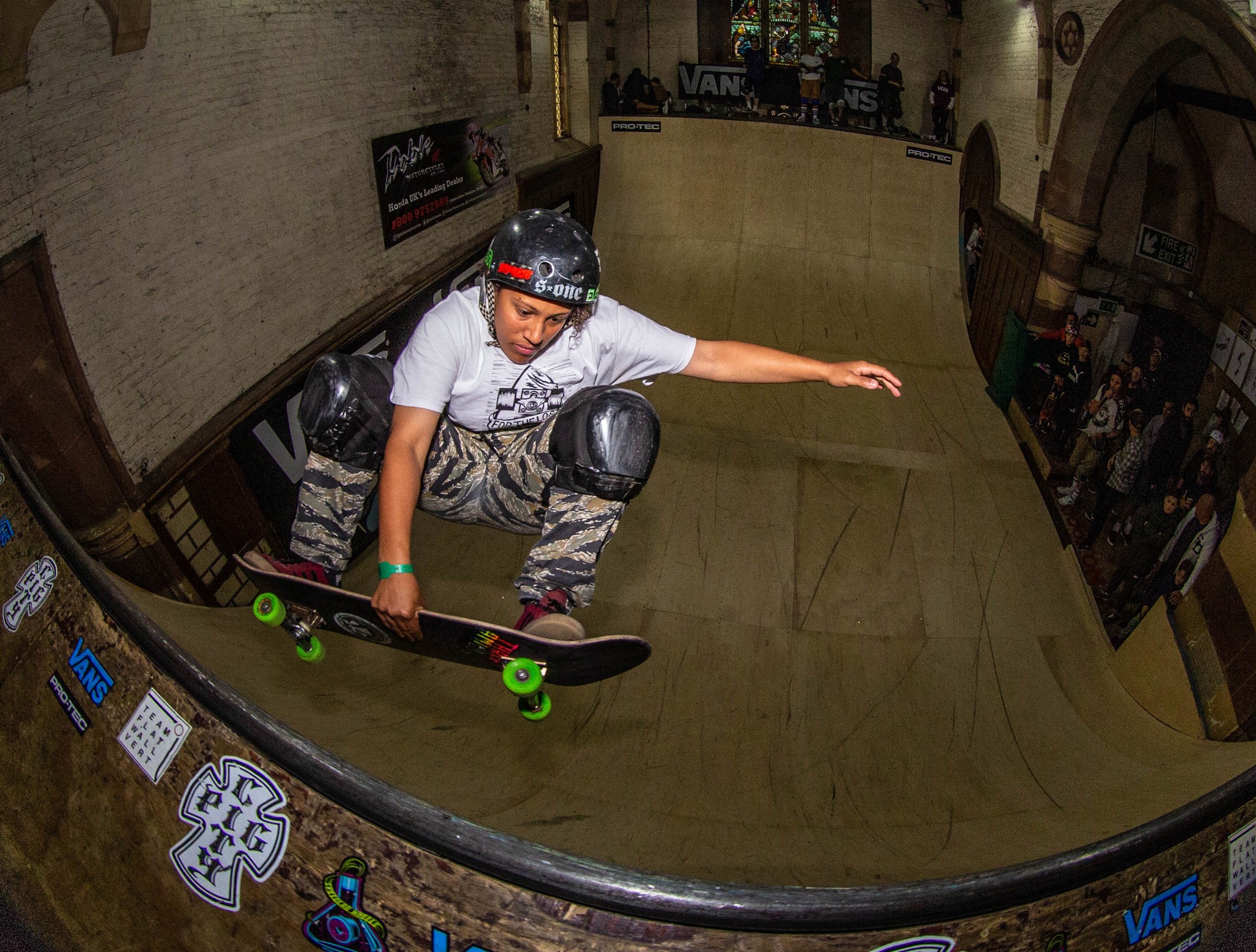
[378,561,416,580]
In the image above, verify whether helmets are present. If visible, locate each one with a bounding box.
[1065,323,1078,335]
[1210,429,1225,444]
[485,208,600,303]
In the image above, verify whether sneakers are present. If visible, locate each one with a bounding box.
[796,116,806,122]
[875,125,890,134]
[514,589,584,642]
[935,136,940,142]
[942,133,948,144]
[750,111,758,114]
[888,126,905,136]
[813,118,820,124]
[663,100,670,115]
[244,551,341,588]
[741,107,751,112]
[832,116,851,126]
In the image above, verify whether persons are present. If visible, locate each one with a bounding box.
[597,35,957,146]
[244,207,902,645]
[466,124,501,170]
[1024,308,1241,637]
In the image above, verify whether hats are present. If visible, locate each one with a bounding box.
[1130,409,1146,429]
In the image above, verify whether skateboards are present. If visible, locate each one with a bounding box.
[231,549,655,724]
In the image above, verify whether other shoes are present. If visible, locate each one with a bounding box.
[1022,406,1080,449]
[1055,459,1072,470]
[1057,485,1119,552]
[1093,581,1143,635]
